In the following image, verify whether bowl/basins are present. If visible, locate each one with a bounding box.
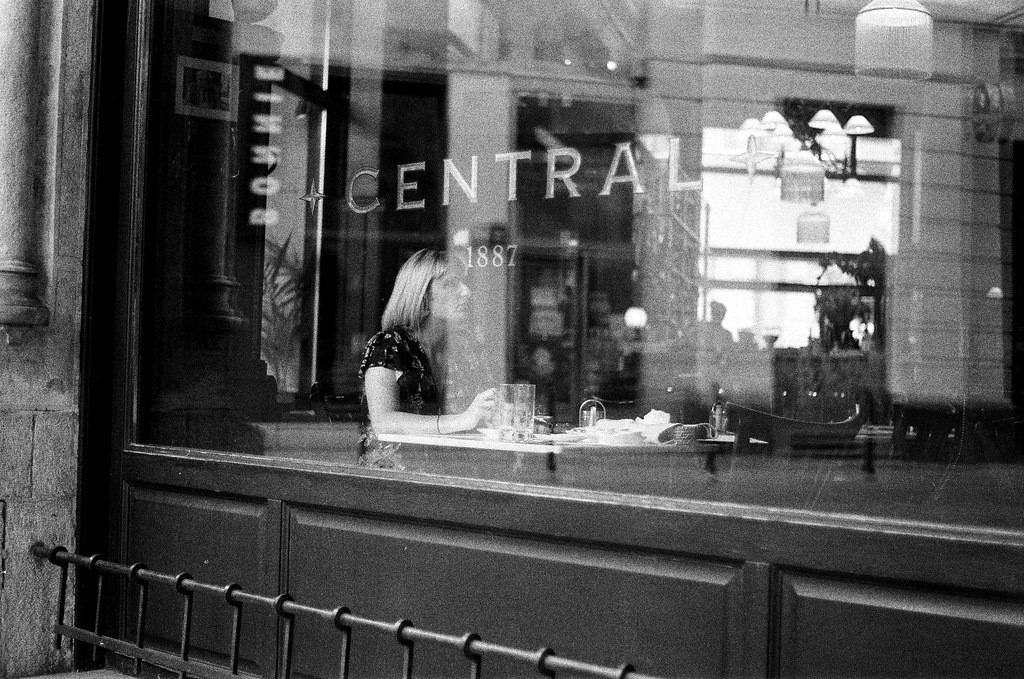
[667,425,699,445]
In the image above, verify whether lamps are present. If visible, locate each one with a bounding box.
[737,117,765,137]
[808,109,842,131]
[760,110,789,130]
[855,0,935,83]
[844,116,874,135]
[796,204,831,245]
[778,150,826,204]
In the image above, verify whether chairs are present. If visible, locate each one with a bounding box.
[723,400,872,509]
[888,404,960,490]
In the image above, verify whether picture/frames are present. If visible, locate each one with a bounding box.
[174,55,241,122]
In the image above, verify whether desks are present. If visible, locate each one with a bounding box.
[377,424,955,511]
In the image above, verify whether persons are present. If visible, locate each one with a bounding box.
[700,303,731,346]
[358,251,515,462]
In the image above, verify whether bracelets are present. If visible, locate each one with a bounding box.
[437,414,442,436]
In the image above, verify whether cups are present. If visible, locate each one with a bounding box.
[582,412,602,427]
[499,383,535,442]
[534,416,552,434]
